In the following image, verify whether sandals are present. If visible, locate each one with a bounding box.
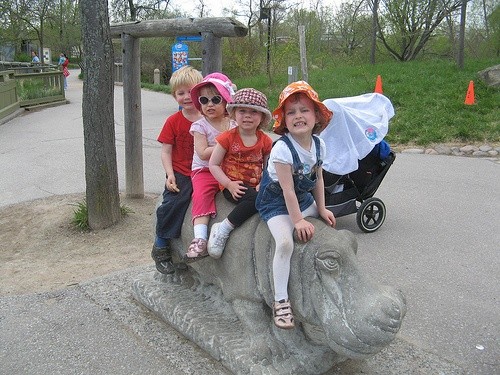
[272,299,295,328]
[186,238,208,257]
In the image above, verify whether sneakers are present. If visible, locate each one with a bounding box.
[151,245,175,274]
[208,223,230,259]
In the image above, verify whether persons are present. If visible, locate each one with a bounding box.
[207,88,274,260]
[183,73,238,264]
[151,64,203,273]
[58,52,69,90]
[255,81,336,329]
[31,51,39,66]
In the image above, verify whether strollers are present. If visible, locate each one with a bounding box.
[312,93,396,233]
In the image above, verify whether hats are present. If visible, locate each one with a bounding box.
[273,81,333,135]
[226,88,272,126]
[190,73,235,114]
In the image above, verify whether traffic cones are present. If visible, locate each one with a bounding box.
[372,75,383,94]
[463,80,475,105]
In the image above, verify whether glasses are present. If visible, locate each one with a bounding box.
[198,95,222,105]
